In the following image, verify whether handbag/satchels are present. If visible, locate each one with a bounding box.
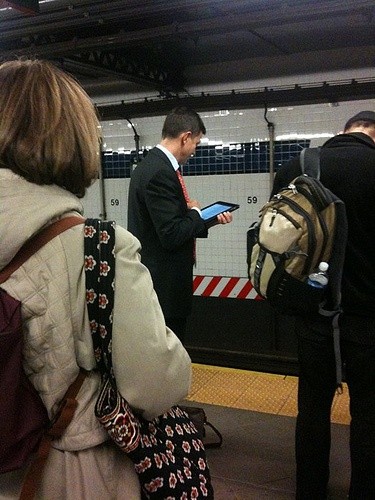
[83,218,215,499]
[177,401,223,449]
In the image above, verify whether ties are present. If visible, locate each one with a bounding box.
[176,168,190,203]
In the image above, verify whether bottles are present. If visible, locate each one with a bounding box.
[307,262,330,289]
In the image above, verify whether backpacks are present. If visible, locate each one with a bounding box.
[0,208,88,500]
[246,145,347,394]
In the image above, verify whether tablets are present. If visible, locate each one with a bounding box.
[197,200,239,224]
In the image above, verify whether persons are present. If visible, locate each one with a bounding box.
[268,111,375,500]
[0,58,191,499]
[126,105,234,347]
